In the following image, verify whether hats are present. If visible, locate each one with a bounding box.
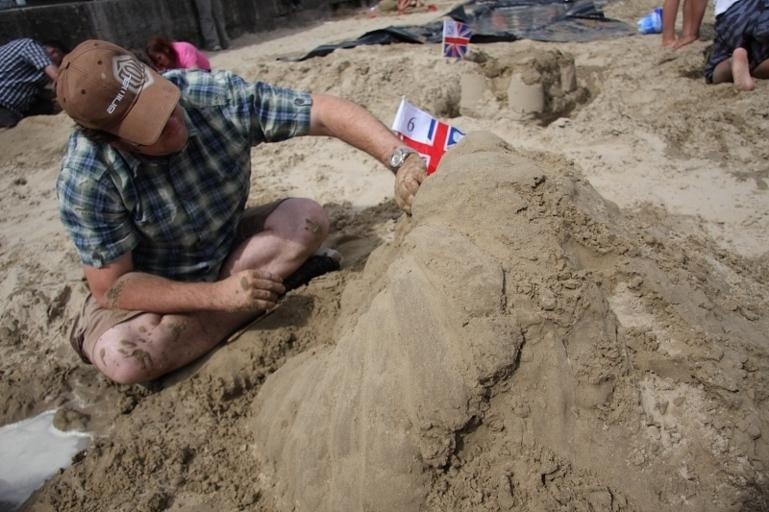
[53,37,182,147]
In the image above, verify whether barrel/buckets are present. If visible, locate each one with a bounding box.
[637,7,663,36]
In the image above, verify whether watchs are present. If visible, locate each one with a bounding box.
[388,146,418,175]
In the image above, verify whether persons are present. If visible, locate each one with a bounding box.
[54,38,431,386]
[143,33,213,76]
[700,1,769,92]
[0,32,72,129]
[659,0,707,57]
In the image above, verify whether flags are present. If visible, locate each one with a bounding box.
[388,95,468,181]
[442,16,472,59]
